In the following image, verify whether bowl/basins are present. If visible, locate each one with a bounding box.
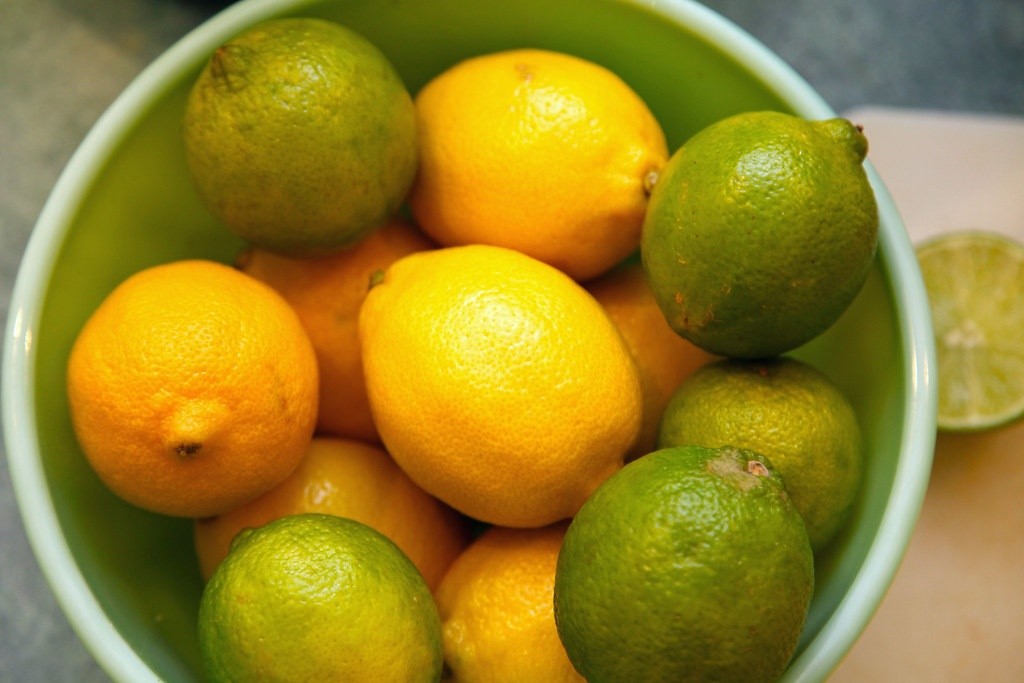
[1,0,940,683]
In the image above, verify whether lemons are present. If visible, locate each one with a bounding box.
[68,16,879,683]
[910,230,1024,433]
